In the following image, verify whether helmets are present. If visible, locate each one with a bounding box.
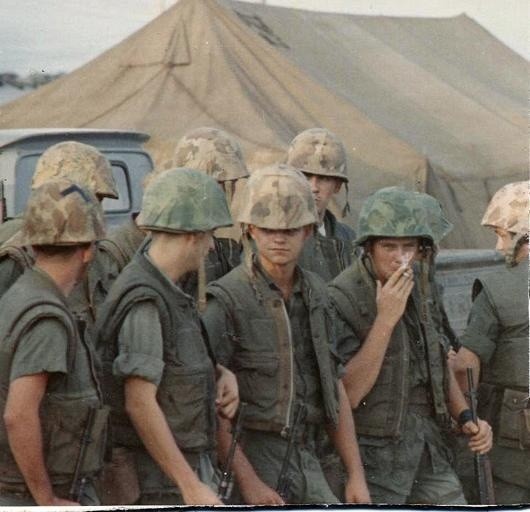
[284,127,349,184]
[417,190,455,246]
[480,181,530,241]
[352,185,435,248]
[235,162,322,230]
[30,139,119,200]
[20,174,107,248]
[136,167,233,234]
[164,127,250,183]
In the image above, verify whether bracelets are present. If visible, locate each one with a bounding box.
[456,408,476,424]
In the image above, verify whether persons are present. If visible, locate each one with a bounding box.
[0,140,125,334]
[287,128,364,285]
[200,161,372,507]
[90,165,242,507]
[327,185,495,507]
[448,178,530,504]
[96,159,177,278]
[174,128,249,301]
[0,178,109,507]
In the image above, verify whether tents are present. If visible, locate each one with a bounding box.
[1,0,530,252]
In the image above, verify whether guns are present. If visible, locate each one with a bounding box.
[275,404,304,503]
[66,405,96,503]
[466,367,495,505]
[216,401,247,505]
